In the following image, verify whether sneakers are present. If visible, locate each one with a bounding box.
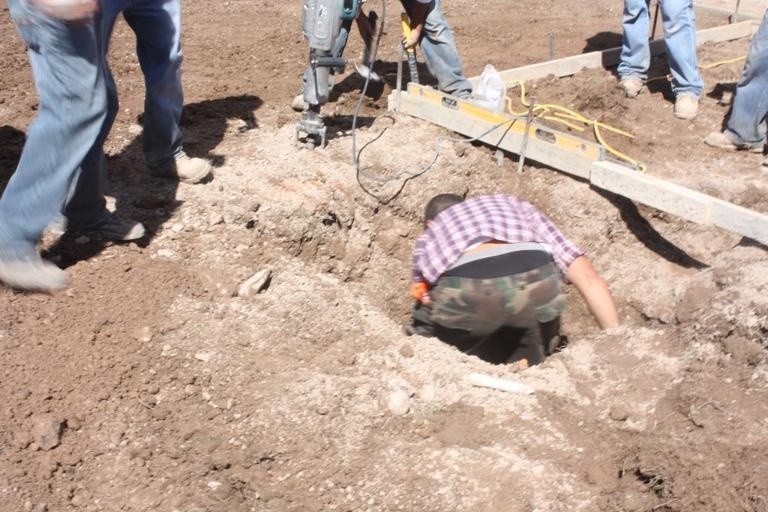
[291,86,332,110]
[451,89,472,102]
[704,132,764,152]
[68,209,145,243]
[144,151,213,184]
[0,255,70,292]
[672,95,698,120]
[619,77,643,98]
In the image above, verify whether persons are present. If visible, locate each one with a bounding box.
[0,0,146,293]
[96,0,211,184]
[616,0,704,119]
[704,7,768,168]
[292,0,474,110]
[410,193,620,368]
